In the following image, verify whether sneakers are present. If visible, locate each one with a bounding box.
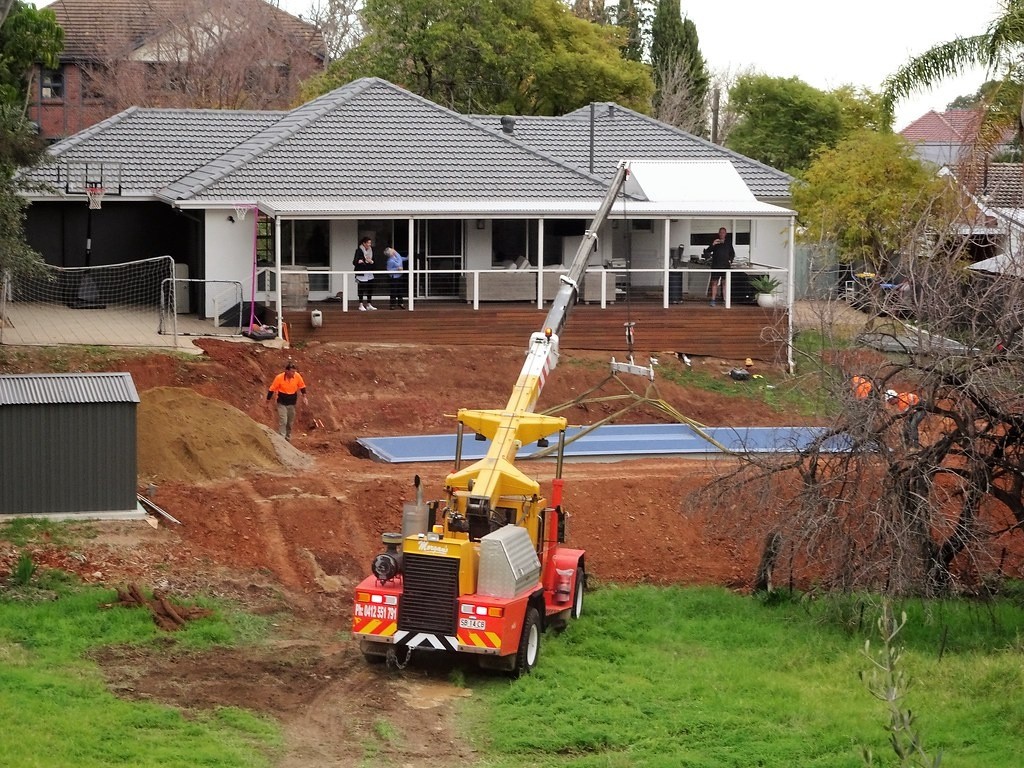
[359,306,367,311]
[367,305,376,310]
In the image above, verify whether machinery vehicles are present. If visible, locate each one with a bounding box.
[349,156,631,681]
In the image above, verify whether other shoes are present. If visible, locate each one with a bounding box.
[389,306,395,310]
[400,303,406,309]
[709,301,716,307]
[721,301,726,307]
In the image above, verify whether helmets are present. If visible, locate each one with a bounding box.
[885,390,898,401]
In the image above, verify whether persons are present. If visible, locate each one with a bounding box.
[884,389,919,413]
[353,237,377,312]
[850,375,877,402]
[267,363,308,443]
[704,228,735,308]
[384,246,408,309]
